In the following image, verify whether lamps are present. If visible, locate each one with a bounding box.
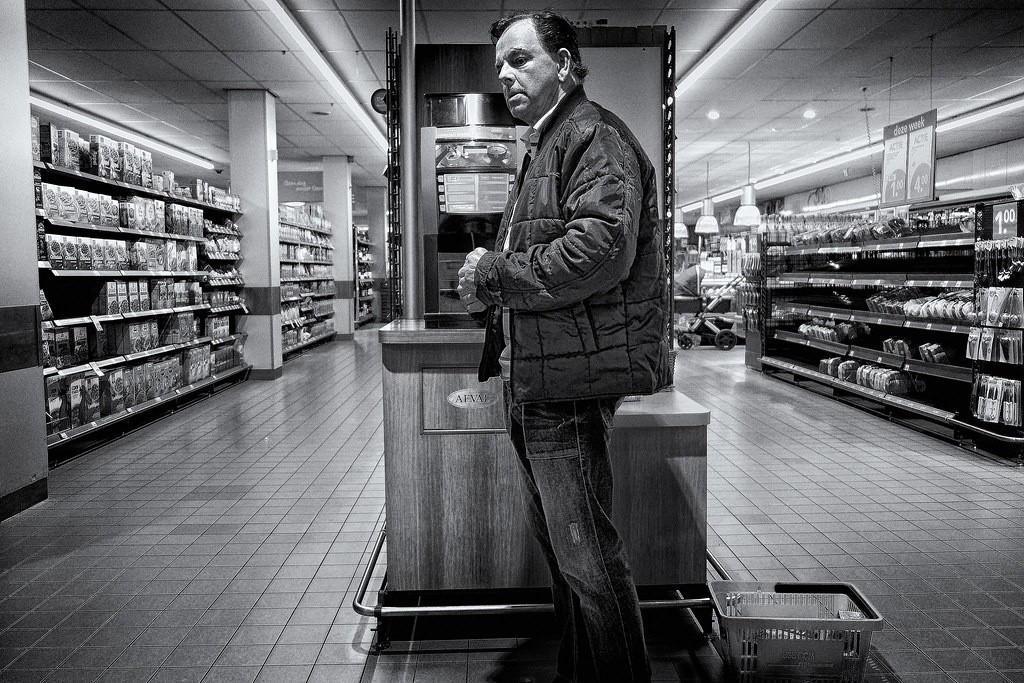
[671,178,688,237]
[733,140,763,228]
[693,161,720,235]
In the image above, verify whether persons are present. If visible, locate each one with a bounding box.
[457,7,673,683]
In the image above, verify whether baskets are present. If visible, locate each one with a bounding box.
[706,580,883,682]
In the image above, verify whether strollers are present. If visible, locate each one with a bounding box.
[668,264,744,351]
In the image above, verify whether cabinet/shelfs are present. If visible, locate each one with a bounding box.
[358,238,375,324]
[33,161,255,448]
[757,230,974,445]
[279,217,337,360]
